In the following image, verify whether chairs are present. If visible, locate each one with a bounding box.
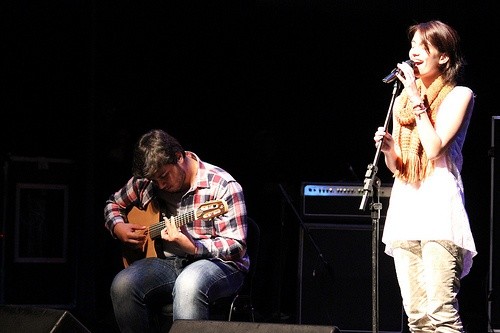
[159,218,260,333]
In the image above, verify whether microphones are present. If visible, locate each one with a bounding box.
[382,60,415,83]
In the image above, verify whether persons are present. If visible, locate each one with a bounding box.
[104,128,250,333]
[374,20,478,333]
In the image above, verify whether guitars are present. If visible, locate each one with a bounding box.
[120,199,229,268]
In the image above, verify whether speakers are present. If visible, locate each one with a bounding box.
[378,225,413,333]
[299,223,376,333]
[169,319,339,333]
[1,306,92,333]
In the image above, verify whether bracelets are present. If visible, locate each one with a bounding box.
[412,100,427,116]
